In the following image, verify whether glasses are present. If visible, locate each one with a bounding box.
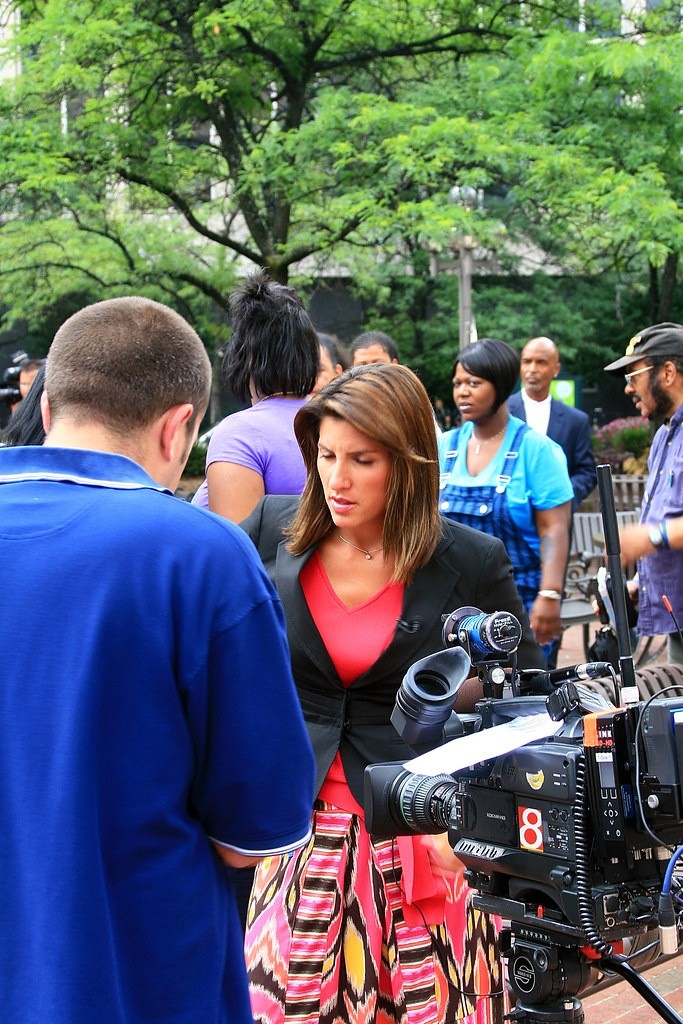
[625,360,680,385]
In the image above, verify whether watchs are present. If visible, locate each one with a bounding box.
[649,523,663,552]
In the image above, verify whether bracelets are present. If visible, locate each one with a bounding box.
[661,520,670,550]
[536,590,562,600]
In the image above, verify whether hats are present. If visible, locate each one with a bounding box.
[603,322,683,371]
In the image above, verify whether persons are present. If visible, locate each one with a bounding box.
[309,333,350,395]
[189,268,321,525]
[243,364,554,1023]
[604,322,683,668]
[349,331,399,368]
[436,338,575,663]
[505,335,598,606]
[0,295,316,1024]
[10,359,42,417]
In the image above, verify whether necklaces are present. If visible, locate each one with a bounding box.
[338,531,399,561]
[472,424,505,454]
[260,391,293,402]
